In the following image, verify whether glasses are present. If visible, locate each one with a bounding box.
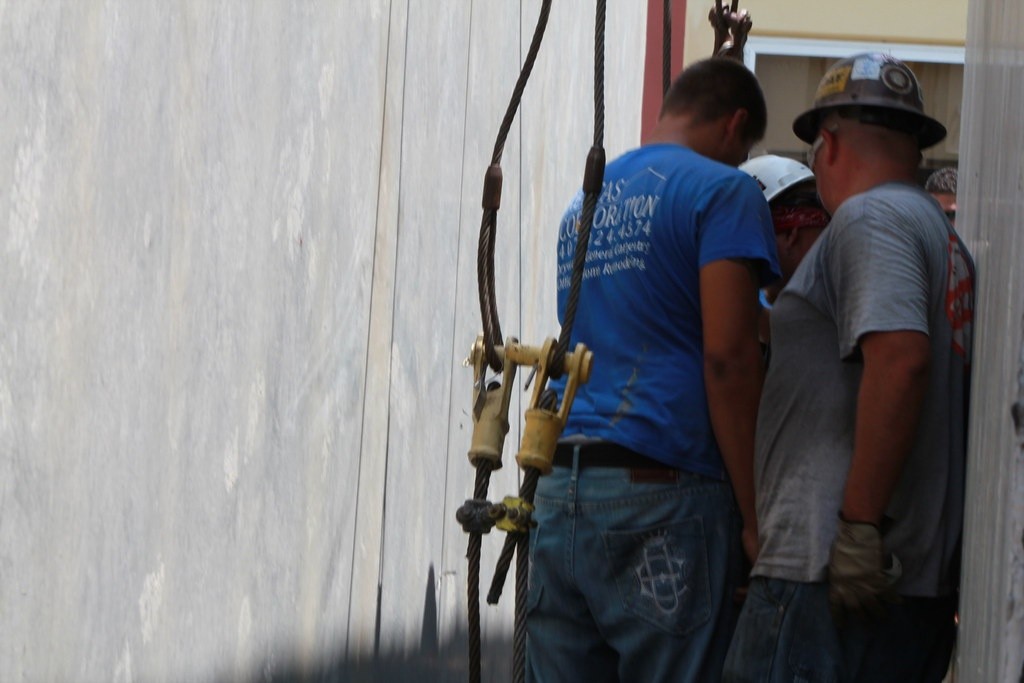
[946,207,957,221]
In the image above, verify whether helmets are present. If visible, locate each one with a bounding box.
[735,154,815,202]
[792,52,946,148]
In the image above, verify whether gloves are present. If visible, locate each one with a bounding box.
[825,521,904,630]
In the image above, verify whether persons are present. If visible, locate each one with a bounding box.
[713,47,979,683]
[525,58,783,683]
[924,167,960,227]
[735,153,833,365]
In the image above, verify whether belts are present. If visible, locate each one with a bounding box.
[551,443,638,467]
